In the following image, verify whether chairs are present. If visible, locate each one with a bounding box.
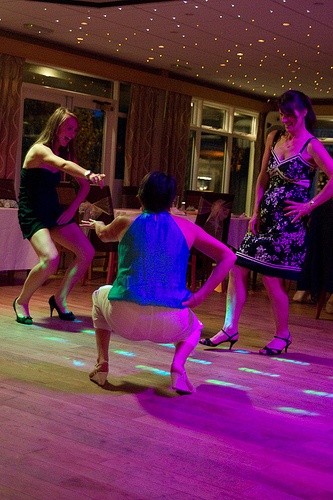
[187,190,235,293]
[71,180,119,286]
[103,185,141,271]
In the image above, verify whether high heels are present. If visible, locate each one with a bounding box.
[13,297,33,324]
[89,361,109,385]
[171,361,196,392]
[259,332,292,356]
[48,295,76,321]
[199,328,239,350]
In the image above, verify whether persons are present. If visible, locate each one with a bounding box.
[80,171,236,393]
[199,91,333,353]
[13,108,106,324]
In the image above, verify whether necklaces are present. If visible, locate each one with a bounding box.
[286,134,293,148]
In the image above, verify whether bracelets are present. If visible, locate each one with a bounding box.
[310,200,314,204]
[85,170,91,177]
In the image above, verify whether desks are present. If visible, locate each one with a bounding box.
[227,215,252,250]
[0,204,38,279]
[106,208,198,286]
